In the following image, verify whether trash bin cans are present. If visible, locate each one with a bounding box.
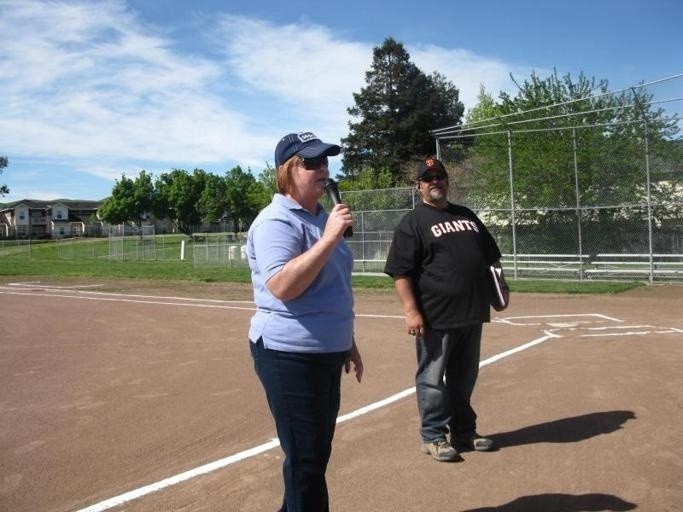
[228,245,247,261]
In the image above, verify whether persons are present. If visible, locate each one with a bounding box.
[245,130,364,512]
[383,158,510,461]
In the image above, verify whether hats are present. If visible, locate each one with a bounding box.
[416,158,445,177]
[274,132,340,168]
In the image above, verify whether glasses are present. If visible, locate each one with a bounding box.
[418,173,446,183]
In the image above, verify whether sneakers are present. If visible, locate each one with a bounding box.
[450,431,496,451]
[423,436,460,463]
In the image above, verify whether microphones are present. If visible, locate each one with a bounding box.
[323,179,354,239]
